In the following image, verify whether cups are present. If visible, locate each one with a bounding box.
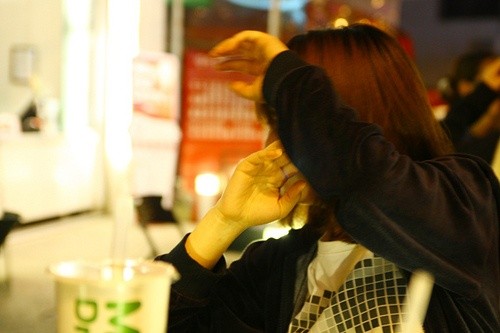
[45,258,179,333]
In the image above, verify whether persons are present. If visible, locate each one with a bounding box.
[441,56,500,165]
[153,24,500,333]
